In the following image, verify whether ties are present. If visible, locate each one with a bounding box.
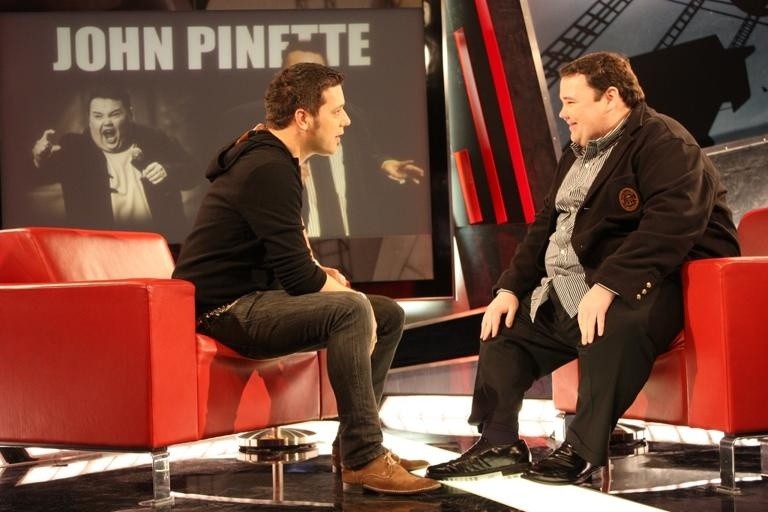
[310,155,346,238]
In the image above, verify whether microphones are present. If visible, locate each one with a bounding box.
[132,147,168,183]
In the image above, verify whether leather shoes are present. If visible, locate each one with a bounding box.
[521,440,602,485]
[332,496,440,511]
[425,436,532,480]
[332,446,441,494]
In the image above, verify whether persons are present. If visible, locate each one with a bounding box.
[213,40,426,239]
[425,50,741,485]
[33,87,203,243]
[171,62,443,497]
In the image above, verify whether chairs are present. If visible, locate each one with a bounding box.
[0,227,340,509]
[551,207,767,496]
